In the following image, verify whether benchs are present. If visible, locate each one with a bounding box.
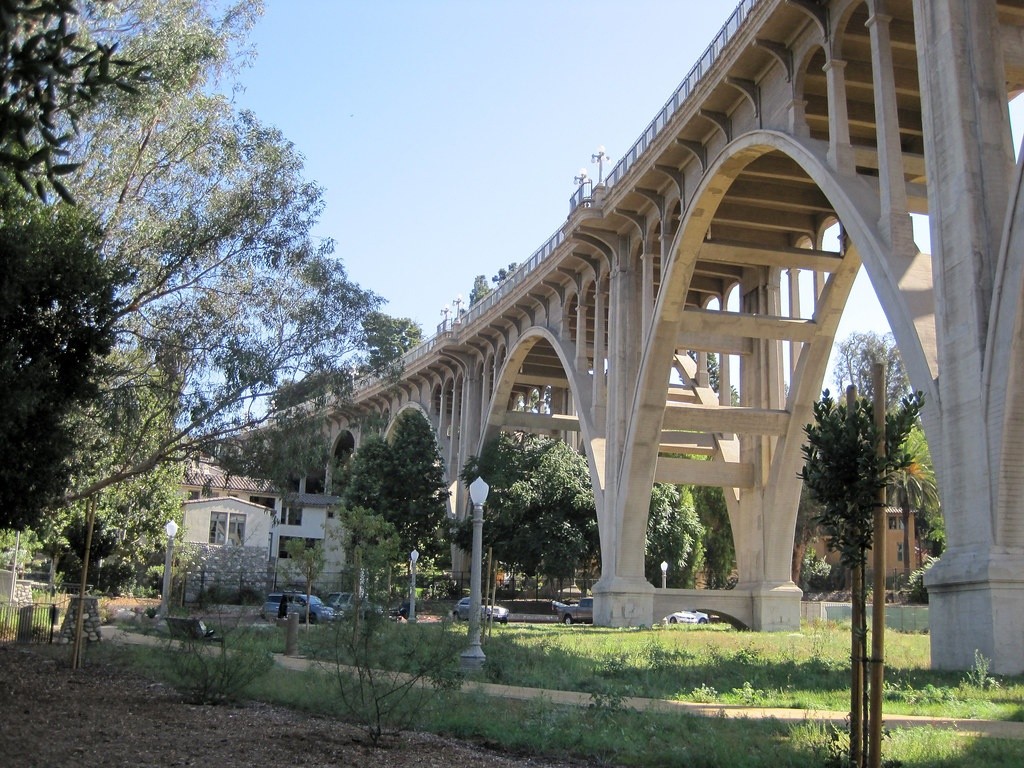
[162,617,225,653]
[61,583,94,595]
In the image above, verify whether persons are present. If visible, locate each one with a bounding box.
[396,605,409,619]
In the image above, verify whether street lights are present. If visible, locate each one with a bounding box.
[457,476,490,674]
[573,168,593,198]
[591,145,611,184]
[439,304,453,320]
[159,519,180,618]
[407,549,419,624]
[660,560,669,588]
[451,296,465,318]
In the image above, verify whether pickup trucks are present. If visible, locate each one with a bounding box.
[556,597,593,625]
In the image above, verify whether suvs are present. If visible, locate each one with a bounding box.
[258,592,336,623]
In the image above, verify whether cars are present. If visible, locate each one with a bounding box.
[665,611,710,625]
[452,596,511,623]
[326,591,402,622]
